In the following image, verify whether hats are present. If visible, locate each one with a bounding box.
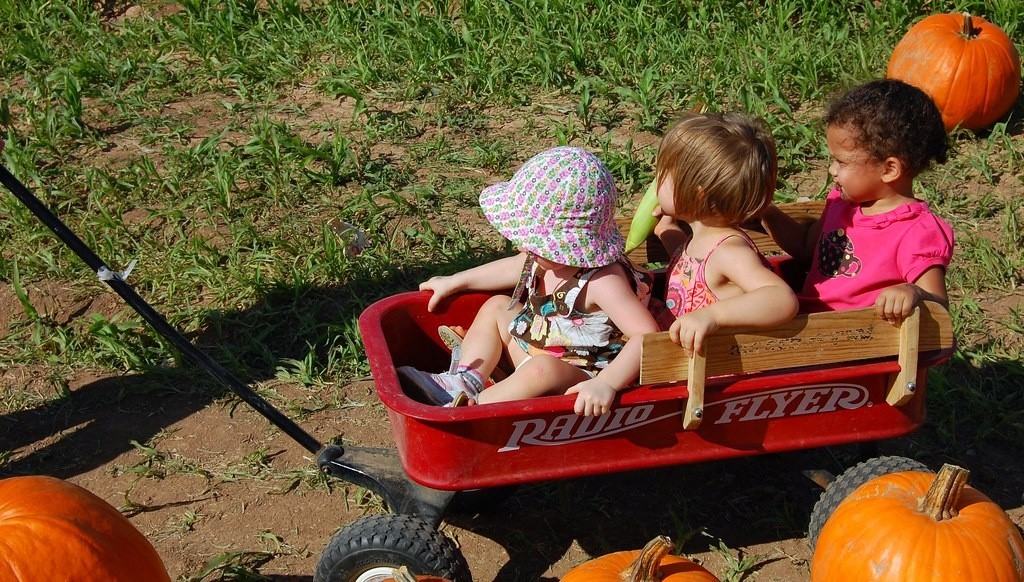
[478,146,628,268]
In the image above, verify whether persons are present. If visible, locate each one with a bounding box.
[759,79,954,324]
[652,112,798,359]
[394,146,661,417]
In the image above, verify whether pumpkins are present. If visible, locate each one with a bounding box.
[887,14,1020,129]
[812,464,1024,581]
[558,536,722,582]
[0,460,169,581]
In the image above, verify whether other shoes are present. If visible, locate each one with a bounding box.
[450,390,476,407]
[394,346,477,406]
[438,322,508,382]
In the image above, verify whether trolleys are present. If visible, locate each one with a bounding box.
[0,154,958,580]
